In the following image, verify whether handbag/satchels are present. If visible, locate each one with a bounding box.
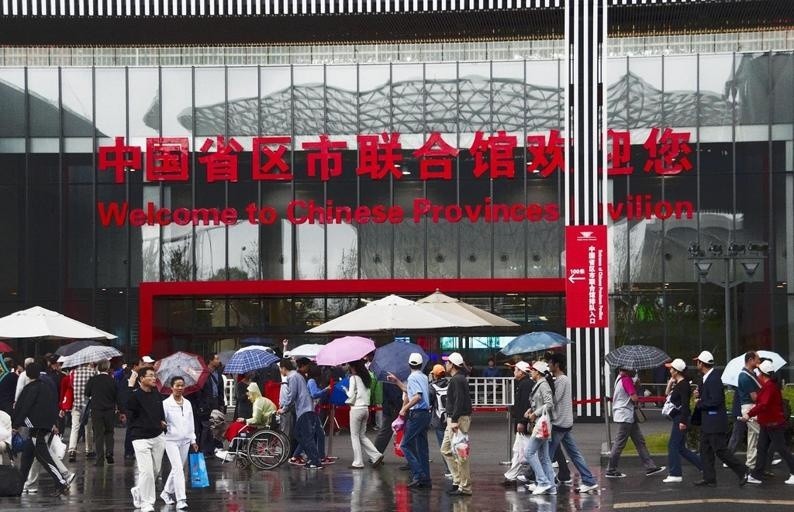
[634,406,647,423]
[0,465,24,497]
[190,453,210,487]
[662,401,679,420]
[210,408,225,430]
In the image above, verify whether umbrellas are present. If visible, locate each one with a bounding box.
[603,342,671,374]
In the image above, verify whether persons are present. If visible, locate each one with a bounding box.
[1,349,793,511]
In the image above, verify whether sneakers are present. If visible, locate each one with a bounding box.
[214,447,236,463]
[289,455,336,470]
[63,439,137,463]
[445,473,472,495]
[694,480,717,487]
[22,488,38,493]
[501,458,598,495]
[605,471,627,478]
[400,463,431,489]
[646,466,666,475]
[663,476,682,482]
[722,459,794,487]
[114,424,124,428]
[131,486,190,512]
[50,473,75,497]
[349,454,384,469]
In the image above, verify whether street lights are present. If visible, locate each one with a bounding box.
[689,242,767,366]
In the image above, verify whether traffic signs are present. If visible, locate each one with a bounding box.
[565,226,609,328]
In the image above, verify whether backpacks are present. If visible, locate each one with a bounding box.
[431,381,450,423]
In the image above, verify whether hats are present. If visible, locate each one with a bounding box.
[756,359,773,376]
[665,358,686,373]
[430,364,445,376]
[511,361,549,374]
[142,356,155,363]
[692,350,715,365]
[409,352,423,366]
[442,352,464,367]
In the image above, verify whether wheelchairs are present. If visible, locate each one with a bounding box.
[221,411,291,470]
